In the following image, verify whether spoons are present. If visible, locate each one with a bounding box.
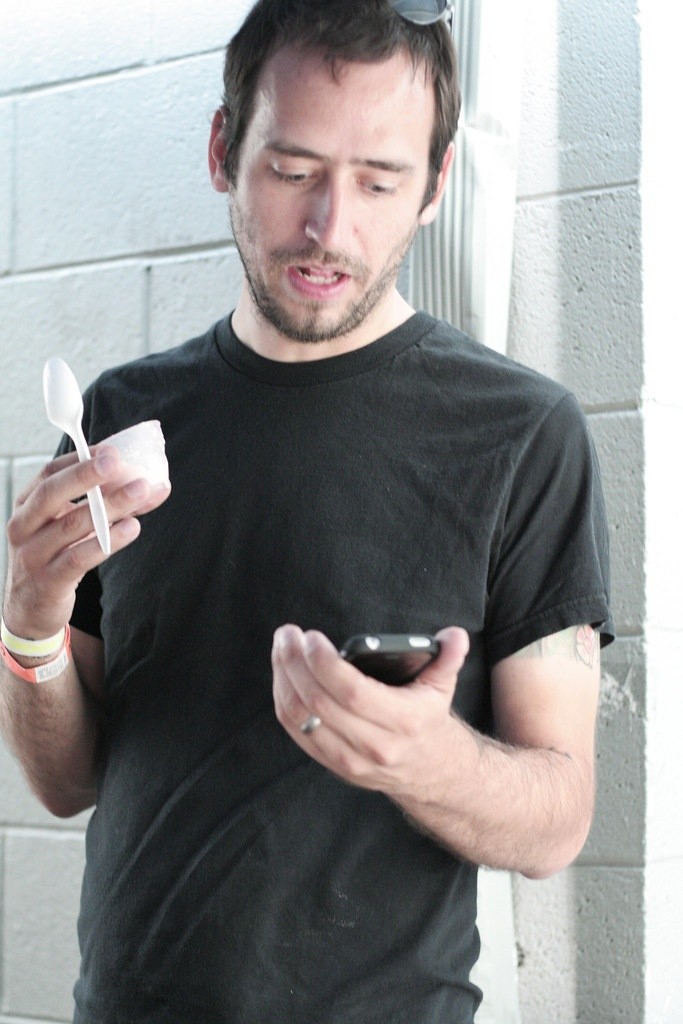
[42,355,111,555]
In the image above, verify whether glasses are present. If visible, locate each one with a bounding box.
[387,0,454,38]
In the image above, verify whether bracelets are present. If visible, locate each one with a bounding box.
[0,625,71,683]
[0,618,65,657]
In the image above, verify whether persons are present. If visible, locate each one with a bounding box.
[0,0,617,1024]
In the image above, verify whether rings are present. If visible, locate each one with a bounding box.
[300,715,321,735]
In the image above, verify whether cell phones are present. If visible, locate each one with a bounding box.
[340,632,438,687]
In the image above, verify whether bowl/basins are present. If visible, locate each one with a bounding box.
[96,419,170,510]
